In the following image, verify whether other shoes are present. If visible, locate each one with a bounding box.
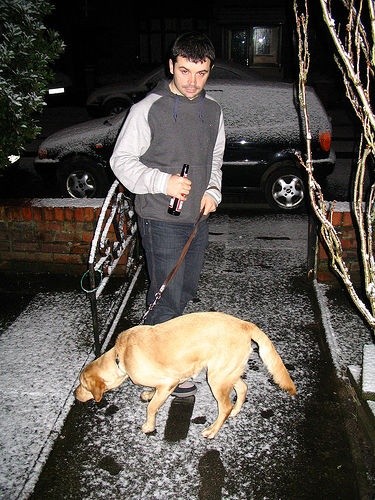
[170,381,197,397]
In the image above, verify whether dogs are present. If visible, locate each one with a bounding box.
[73,310,298,441]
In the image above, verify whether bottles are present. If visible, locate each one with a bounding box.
[168,164,189,216]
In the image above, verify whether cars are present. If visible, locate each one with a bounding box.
[83,56,261,118]
[32,76,337,215]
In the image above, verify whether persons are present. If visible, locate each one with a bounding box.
[110,32,225,398]
